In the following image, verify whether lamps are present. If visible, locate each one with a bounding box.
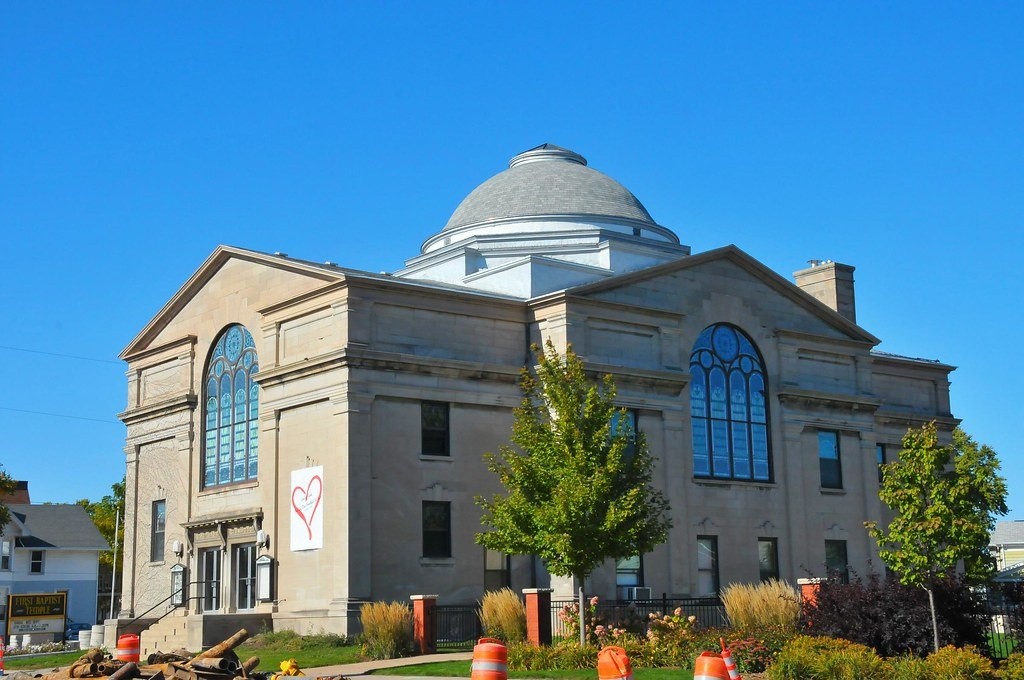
[173,540,183,556]
[255,529,269,550]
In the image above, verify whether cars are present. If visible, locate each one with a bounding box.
[65,622,92,641]
